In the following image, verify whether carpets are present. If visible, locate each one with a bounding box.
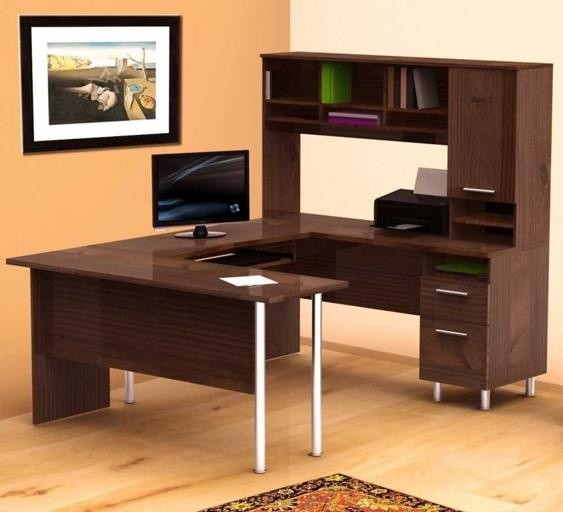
[199,473,462,512]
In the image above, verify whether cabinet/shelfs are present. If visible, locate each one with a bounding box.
[261,52,552,411]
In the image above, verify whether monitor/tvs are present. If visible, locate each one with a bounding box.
[152,150,249,239]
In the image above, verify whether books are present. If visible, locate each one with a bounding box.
[320,62,353,106]
[327,109,382,120]
[433,259,488,278]
[327,115,382,128]
[386,65,440,110]
[265,69,295,101]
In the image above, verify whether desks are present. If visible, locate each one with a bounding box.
[7,217,348,475]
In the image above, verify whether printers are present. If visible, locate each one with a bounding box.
[370,168,448,235]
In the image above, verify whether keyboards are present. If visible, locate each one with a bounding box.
[206,253,280,266]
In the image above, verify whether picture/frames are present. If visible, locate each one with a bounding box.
[19,15,181,152]
